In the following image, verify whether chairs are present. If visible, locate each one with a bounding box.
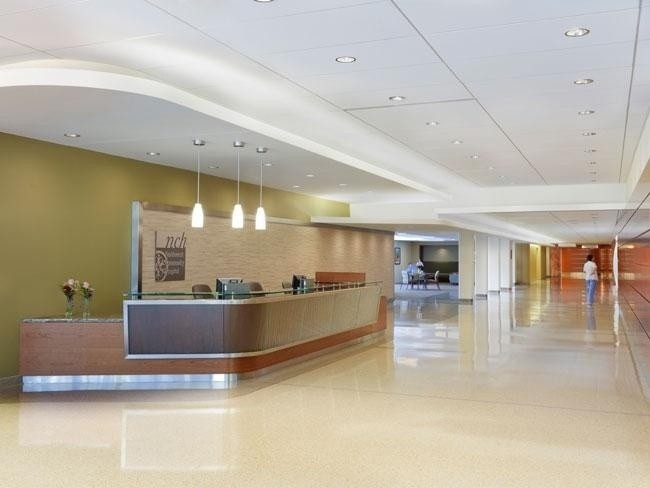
[192,285,215,299]
[400,270,441,291]
[249,282,265,297]
[282,281,292,294]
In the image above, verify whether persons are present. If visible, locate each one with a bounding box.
[583,254,600,306]
[416,258,425,270]
[406,262,418,273]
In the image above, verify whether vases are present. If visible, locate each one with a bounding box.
[65,296,92,319]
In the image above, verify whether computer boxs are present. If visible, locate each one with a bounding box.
[222,283,251,298]
[300,279,315,293]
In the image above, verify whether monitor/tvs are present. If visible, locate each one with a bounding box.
[292,274,306,288]
[216,278,243,299]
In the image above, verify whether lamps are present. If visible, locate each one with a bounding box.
[191,139,267,229]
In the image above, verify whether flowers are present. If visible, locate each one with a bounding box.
[58,279,97,303]
